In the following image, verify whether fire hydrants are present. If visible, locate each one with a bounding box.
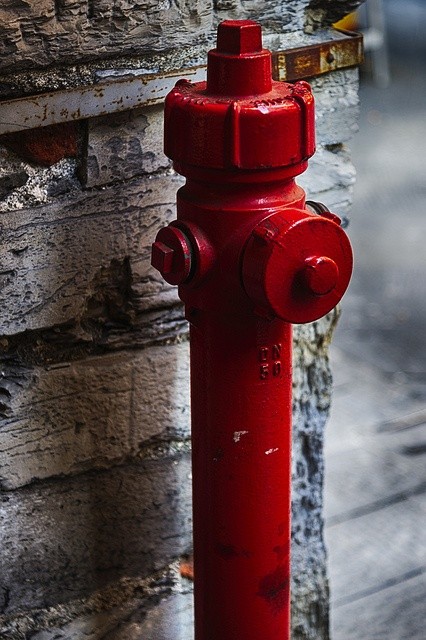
[150,21,353,640]
